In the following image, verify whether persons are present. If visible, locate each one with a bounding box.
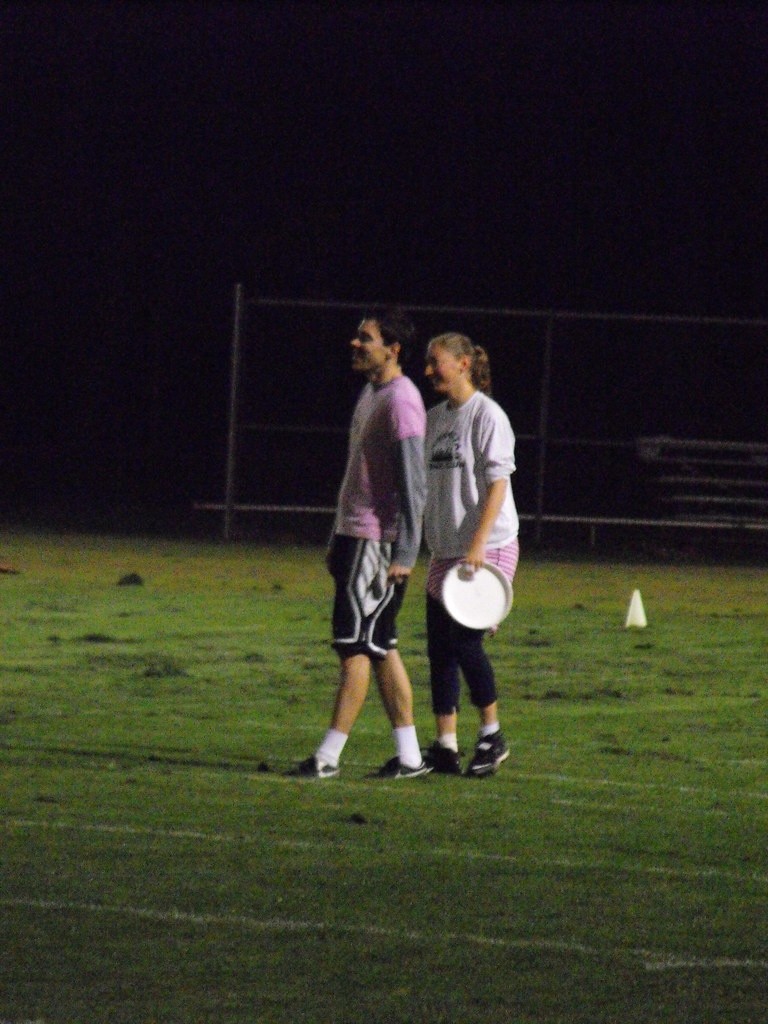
[292,301,431,783]
[421,333,520,777]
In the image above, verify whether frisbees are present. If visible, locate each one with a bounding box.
[442,560,514,631]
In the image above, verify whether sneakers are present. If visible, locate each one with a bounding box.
[369,755,428,781]
[468,726,510,779]
[284,753,342,781]
[420,740,466,778]
